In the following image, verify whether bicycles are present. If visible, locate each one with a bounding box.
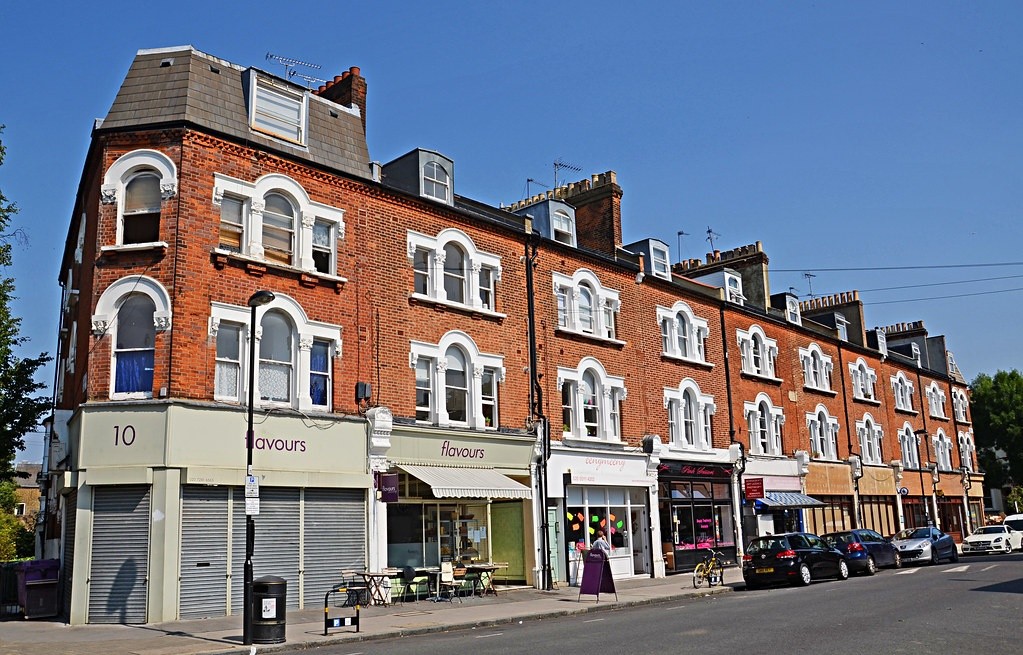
[691,547,725,589]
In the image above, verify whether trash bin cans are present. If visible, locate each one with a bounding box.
[15,558,62,620]
[251,575,288,644]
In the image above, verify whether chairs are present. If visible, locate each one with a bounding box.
[342,570,366,610]
[401,566,430,604]
[439,562,465,604]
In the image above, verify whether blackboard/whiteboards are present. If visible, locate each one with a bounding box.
[579,548,617,595]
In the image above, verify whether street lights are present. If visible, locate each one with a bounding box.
[241,289,275,645]
[913,429,928,527]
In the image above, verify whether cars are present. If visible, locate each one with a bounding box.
[961,524,1023,555]
[890,526,959,566]
[819,528,903,577]
[742,532,849,590]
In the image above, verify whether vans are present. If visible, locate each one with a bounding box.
[1002,514,1023,551]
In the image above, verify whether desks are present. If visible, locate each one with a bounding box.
[464,566,508,598]
[358,573,397,607]
[383,567,439,605]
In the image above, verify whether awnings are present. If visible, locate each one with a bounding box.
[758,491,828,510]
[397,464,532,500]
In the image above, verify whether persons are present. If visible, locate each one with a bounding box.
[592,531,610,552]
